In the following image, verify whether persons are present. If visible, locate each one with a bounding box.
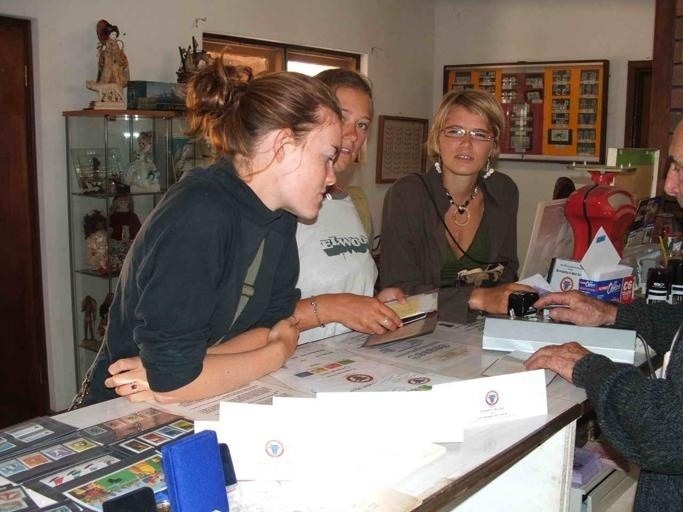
[84,20,129,111]
[107,195,140,242]
[297,68,407,347]
[122,131,161,193]
[522,110,683,512]
[377,87,537,323]
[80,295,97,341]
[96,292,113,337]
[63,43,343,411]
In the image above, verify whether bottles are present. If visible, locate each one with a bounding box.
[645,259,682,304]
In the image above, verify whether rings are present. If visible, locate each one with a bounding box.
[131,382,137,393]
[381,317,388,325]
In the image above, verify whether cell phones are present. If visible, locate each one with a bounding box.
[401,312,427,325]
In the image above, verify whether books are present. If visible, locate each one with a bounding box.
[481,319,637,364]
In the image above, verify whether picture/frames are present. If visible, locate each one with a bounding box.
[443,60,609,165]
[520,197,574,280]
[375,115,427,184]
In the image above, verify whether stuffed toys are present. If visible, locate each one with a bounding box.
[84,207,127,272]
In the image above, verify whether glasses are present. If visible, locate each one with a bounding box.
[443,126,491,142]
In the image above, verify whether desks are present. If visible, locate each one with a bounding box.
[0,312,664,512]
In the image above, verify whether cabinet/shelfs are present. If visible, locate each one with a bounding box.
[61,111,198,394]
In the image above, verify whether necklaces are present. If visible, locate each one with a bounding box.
[442,184,483,227]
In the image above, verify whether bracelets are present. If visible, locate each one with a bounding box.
[309,296,325,329]
